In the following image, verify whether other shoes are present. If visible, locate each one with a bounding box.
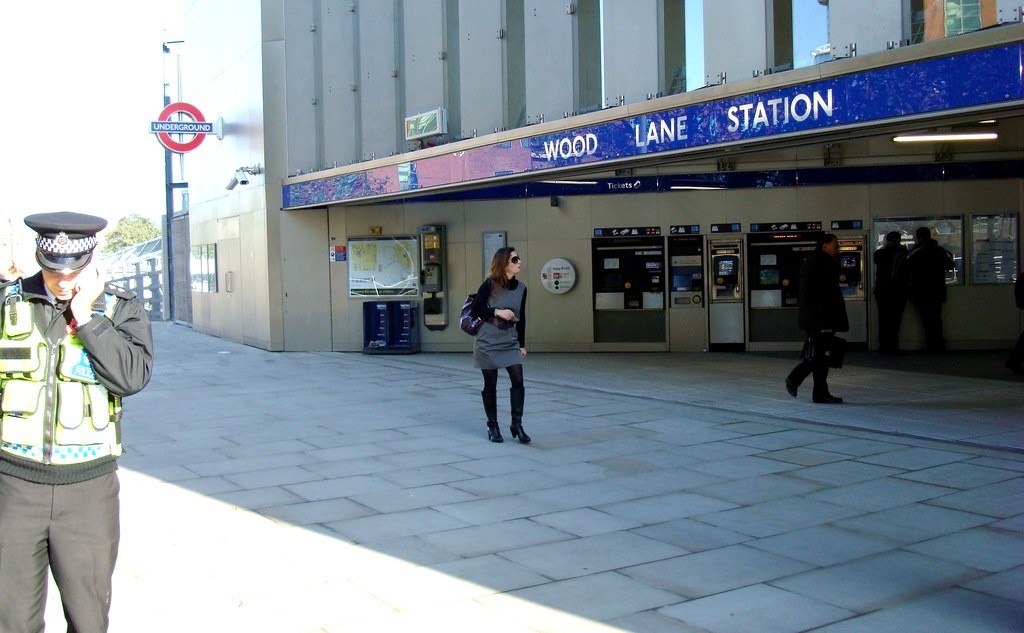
[813,393,842,404]
[786,381,797,398]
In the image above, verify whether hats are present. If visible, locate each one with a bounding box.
[24,212,107,275]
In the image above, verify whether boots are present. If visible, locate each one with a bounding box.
[510,387,531,442]
[481,390,504,443]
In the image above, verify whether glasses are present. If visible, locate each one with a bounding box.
[506,255,522,264]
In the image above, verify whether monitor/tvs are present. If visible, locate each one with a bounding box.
[604,274,623,288]
[719,260,733,275]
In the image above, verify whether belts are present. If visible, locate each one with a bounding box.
[485,316,514,330]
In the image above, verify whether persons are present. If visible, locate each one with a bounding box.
[785,233,850,404]
[905,227,956,356]
[5,257,26,282]
[0,212,154,633]
[873,231,910,358]
[1004,273,1024,377]
[473,247,531,444]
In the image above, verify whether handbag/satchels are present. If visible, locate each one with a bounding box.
[460,296,486,336]
[800,331,846,369]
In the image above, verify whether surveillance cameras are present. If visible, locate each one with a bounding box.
[225,179,238,190]
[236,172,249,185]
